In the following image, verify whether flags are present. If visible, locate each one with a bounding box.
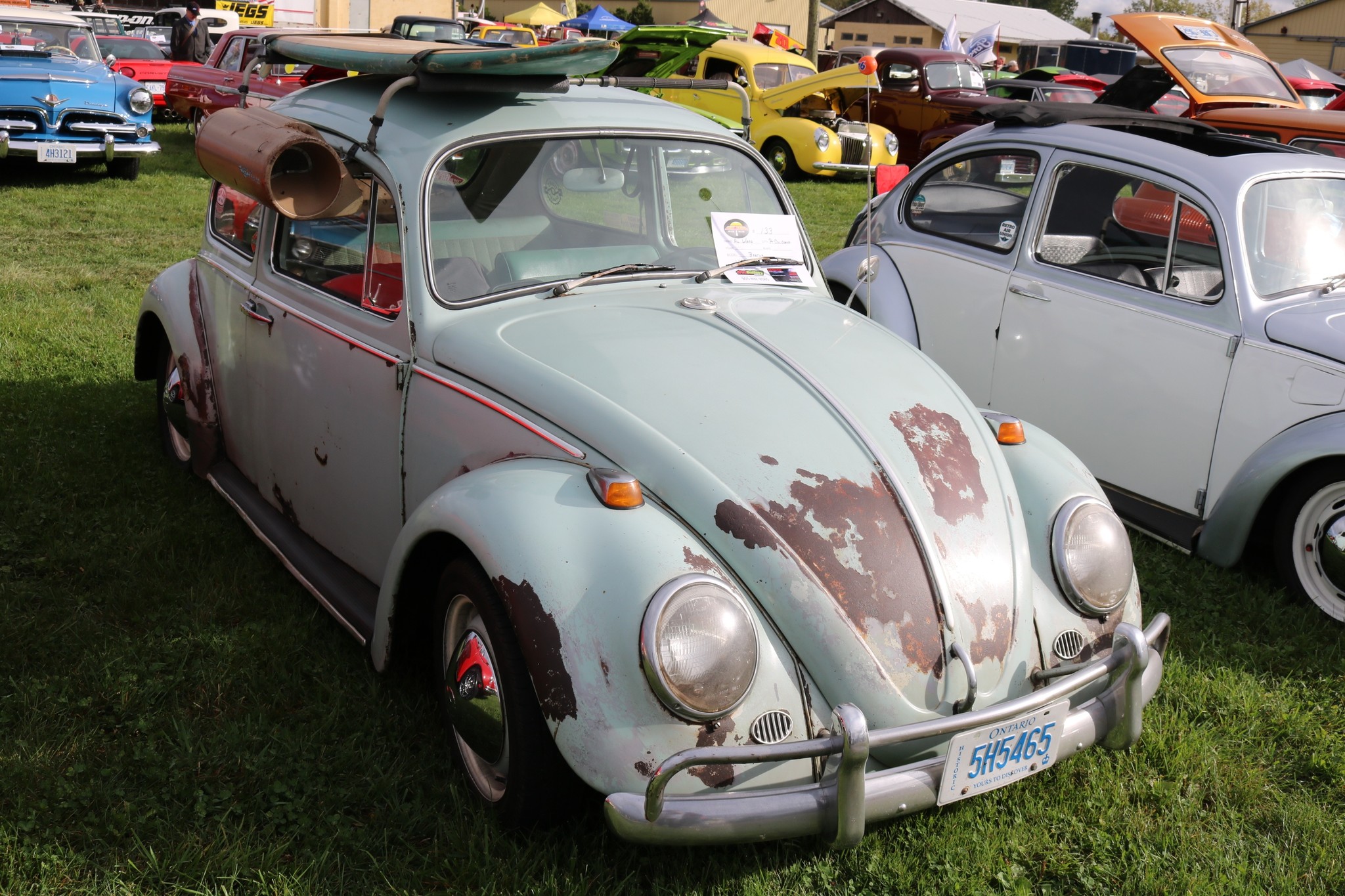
[938,14,1000,63]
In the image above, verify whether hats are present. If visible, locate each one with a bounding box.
[1005,60,1017,67]
[187,1,201,16]
[77,0,85,4]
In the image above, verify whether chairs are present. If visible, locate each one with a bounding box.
[1142,266,1224,295]
[130,47,150,58]
[494,245,660,286]
[1072,262,1149,287]
[997,233,1113,264]
[370,258,487,313]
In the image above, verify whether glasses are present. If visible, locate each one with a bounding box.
[1007,66,1011,69]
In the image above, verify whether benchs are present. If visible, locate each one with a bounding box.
[299,216,554,287]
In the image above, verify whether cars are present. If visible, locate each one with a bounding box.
[1110,12,1343,284]
[819,102,1345,634]
[133,75,1172,854]
[392,16,1345,186]
[0,8,349,182]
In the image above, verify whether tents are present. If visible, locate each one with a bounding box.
[677,9,749,43]
[559,5,637,40]
[503,2,571,40]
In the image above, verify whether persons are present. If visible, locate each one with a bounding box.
[990,56,1022,77]
[517,22,556,44]
[171,2,211,65]
[72,0,109,24]
